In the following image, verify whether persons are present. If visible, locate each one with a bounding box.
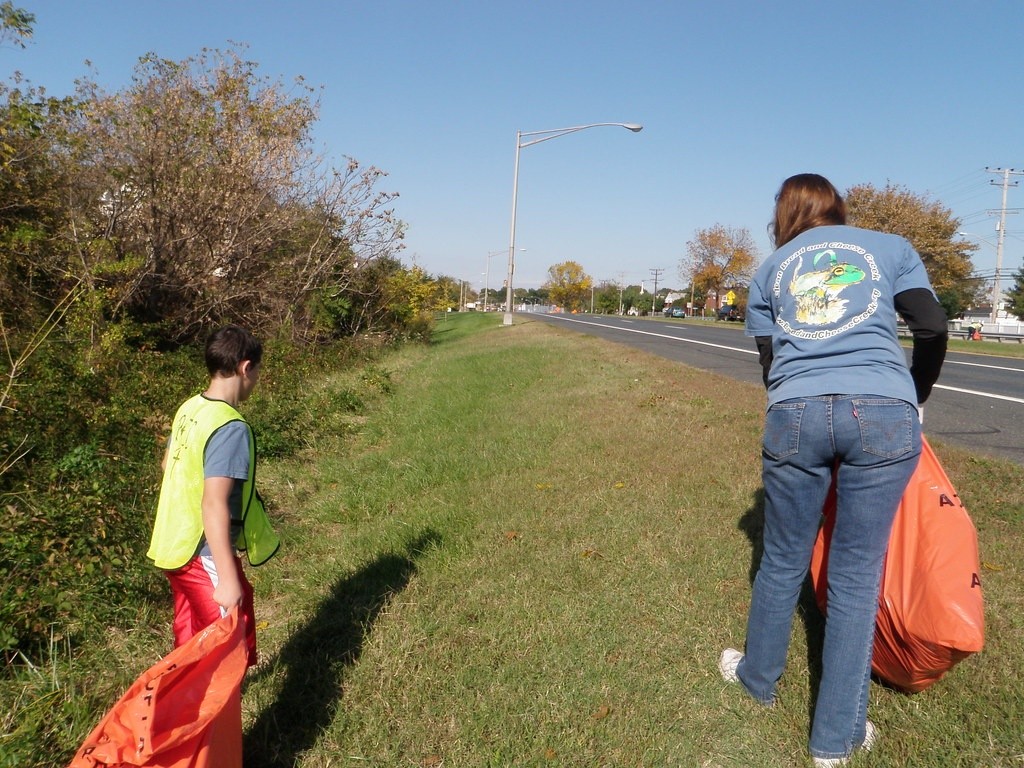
[718,173,949,768]
[967,323,983,340]
[145,324,280,667]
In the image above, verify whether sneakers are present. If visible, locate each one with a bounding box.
[719,648,744,684]
[813,721,877,768]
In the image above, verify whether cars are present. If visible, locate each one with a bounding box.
[664,307,685,319]
[719,305,745,322]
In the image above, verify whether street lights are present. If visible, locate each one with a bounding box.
[502,122,644,326]
[459,273,486,312]
[959,232,1003,323]
[484,249,526,312]
